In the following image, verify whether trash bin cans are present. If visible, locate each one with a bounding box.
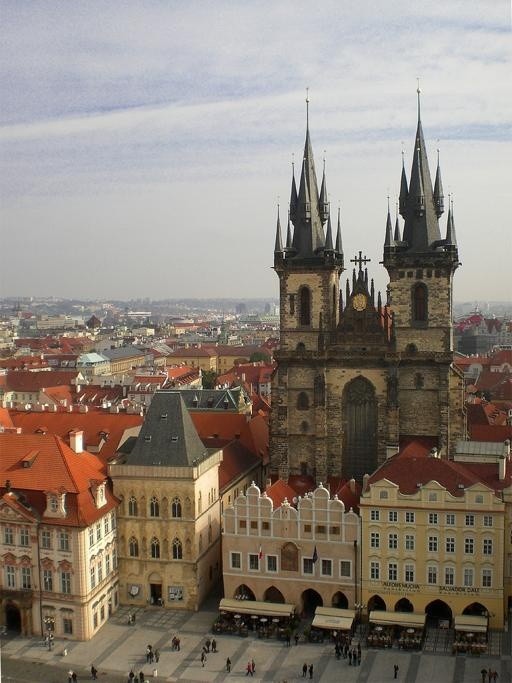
[62,649,67,656]
[152,669,158,677]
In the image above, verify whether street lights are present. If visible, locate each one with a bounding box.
[355,602,367,634]
[43,618,56,651]
[482,611,496,643]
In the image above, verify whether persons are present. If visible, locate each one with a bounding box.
[487,667,493,683]
[91,663,94,676]
[155,648,160,662]
[139,670,145,683]
[206,638,211,652]
[50,632,56,645]
[93,668,98,680]
[66,669,72,683]
[251,659,256,672]
[149,649,154,664]
[146,646,150,662]
[309,663,313,678]
[302,663,307,676]
[480,668,487,682]
[134,675,139,683]
[201,647,208,667]
[129,669,134,683]
[175,638,181,651]
[393,663,399,678]
[212,639,216,652]
[63,648,68,656]
[333,637,362,666]
[492,669,498,683]
[245,661,253,675]
[72,671,78,683]
[227,656,231,672]
[172,636,177,649]
[295,633,298,644]
[287,634,291,646]
[44,632,49,647]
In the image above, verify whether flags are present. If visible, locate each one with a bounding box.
[312,546,318,563]
[258,544,264,560]
